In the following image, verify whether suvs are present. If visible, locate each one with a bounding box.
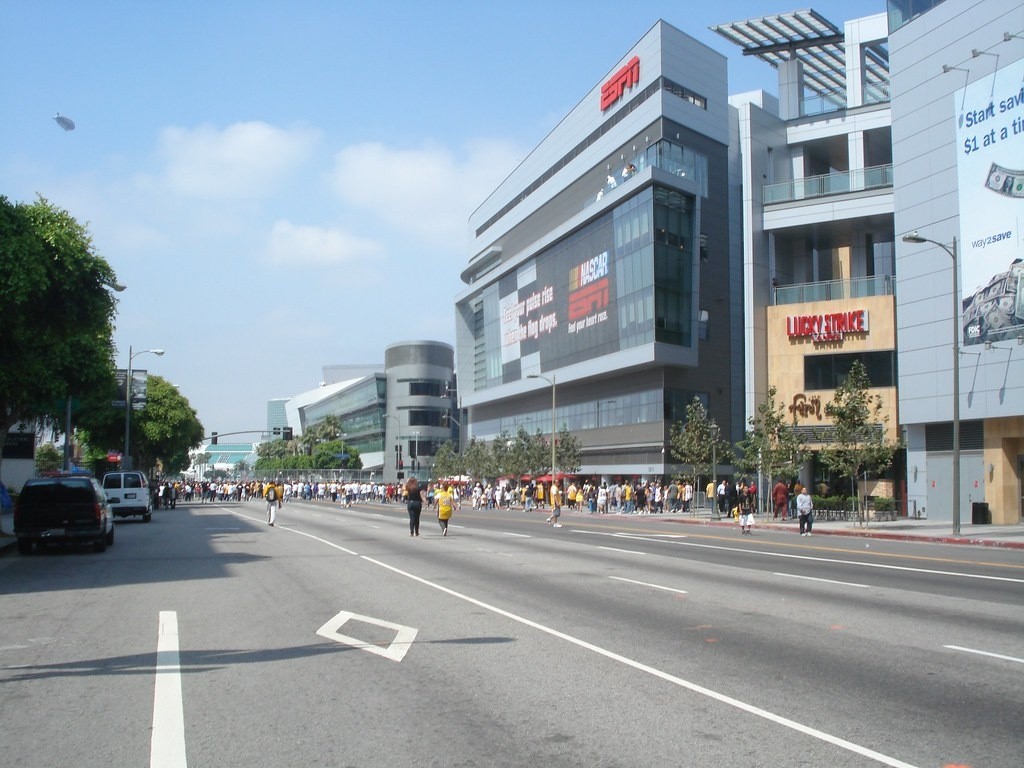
[13,471,114,553]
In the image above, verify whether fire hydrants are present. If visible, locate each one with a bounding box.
[734,507,740,522]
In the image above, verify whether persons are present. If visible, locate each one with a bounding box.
[402,476,427,536]
[566,480,692,515]
[798,487,814,537]
[738,485,755,535]
[425,483,440,508]
[163,484,179,510]
[772,478,803,520]
[546,480,562,527]
[449,481,545,512]
[263,480,282,526]
[433,484,459,535]
[706,481,713,512]
[277,480,407,508]
[176,481,269,502]
[717,479,757,514]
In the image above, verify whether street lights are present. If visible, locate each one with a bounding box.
[526,372,557,483]
[337,432,347,455]
[709,423,722,522]
[123,344,165,470]
[904,230,962,535]
[412,431,420,471]
[440,394,463,487]
[382,413,401,471]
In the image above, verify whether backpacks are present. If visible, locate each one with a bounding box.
[267,488,275,501]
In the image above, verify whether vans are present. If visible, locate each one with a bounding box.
[102,471,153,523]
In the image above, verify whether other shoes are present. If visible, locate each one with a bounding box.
[547,518,551,524]
[415,533,420,536]
[443,528,447,536]
[800,533,805,536]
[741,531,745,534]
[746,530,752,536]
[410,534,413,537]
[554,523,562,527]
[807,532,812,537]
